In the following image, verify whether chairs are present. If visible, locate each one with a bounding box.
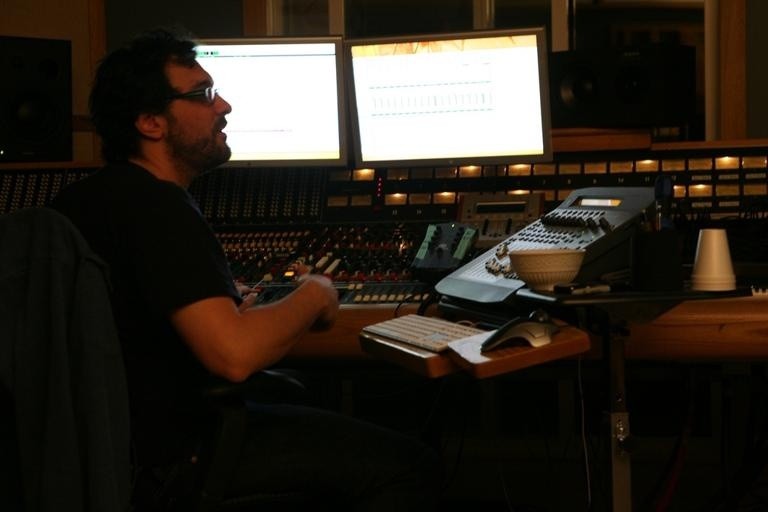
[0,206,336,512]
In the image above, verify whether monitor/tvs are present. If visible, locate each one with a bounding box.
[346,27,553,169]
[173,37,346,168]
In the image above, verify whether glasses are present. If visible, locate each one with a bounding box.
[176,86,221,104]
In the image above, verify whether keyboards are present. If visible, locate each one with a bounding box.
[362,314,486,354]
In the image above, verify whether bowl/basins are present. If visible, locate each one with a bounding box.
[507,249,586,290]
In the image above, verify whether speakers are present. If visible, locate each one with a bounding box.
[0,35,73,163]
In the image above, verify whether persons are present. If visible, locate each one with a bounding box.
[47,30,434,510]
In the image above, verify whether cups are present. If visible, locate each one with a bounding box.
[691,226,735,292]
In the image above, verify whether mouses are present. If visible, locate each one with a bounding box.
[480,307,563,353]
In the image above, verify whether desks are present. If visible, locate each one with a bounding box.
[281,297,768,512]
